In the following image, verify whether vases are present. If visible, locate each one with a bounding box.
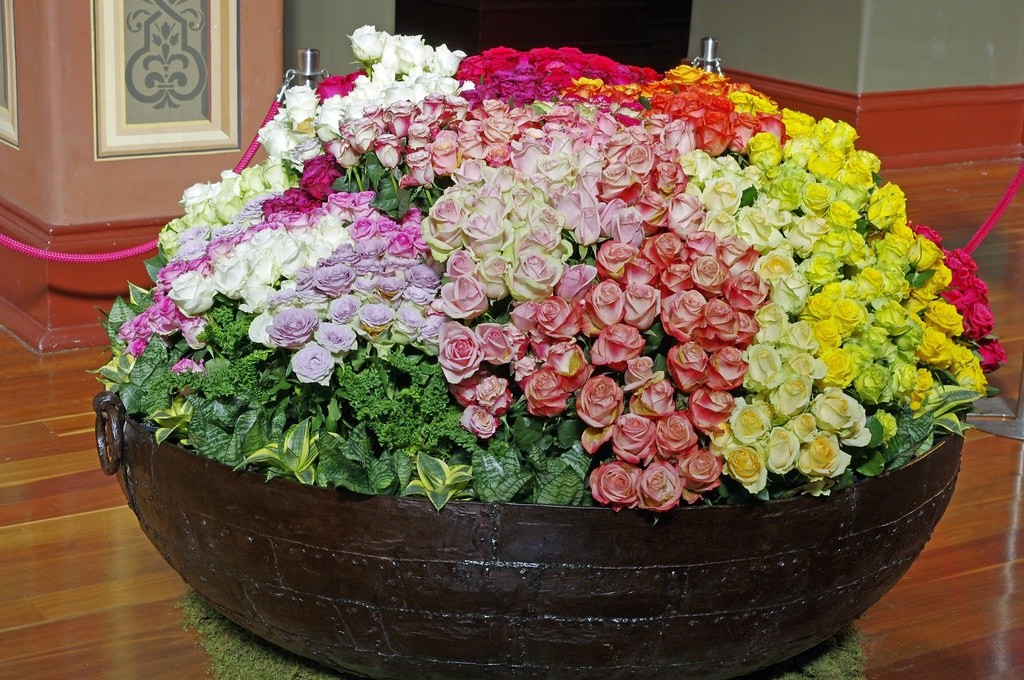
[91,384,973,680]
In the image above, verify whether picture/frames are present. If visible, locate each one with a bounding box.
[0,0,20,151]
[89,0,240,162]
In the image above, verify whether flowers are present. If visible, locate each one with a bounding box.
[79,20,1013,518]
